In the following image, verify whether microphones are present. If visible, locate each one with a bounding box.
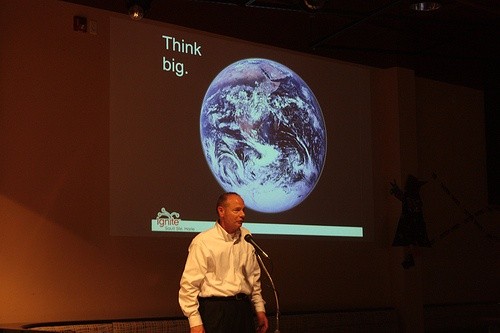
[244,234,269,260]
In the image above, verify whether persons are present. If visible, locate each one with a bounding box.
[178,192,268,333]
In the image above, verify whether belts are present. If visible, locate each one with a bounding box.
[208,294,248,301]
